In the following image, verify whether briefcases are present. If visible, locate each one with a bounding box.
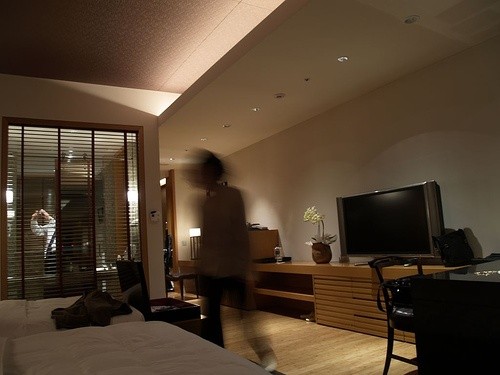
[115,259,201,322]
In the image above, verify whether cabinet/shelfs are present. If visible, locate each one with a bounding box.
[252,260,474,343]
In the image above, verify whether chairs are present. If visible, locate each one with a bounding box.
[369,254,426,375]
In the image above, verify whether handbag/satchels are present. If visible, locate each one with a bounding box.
[432,229,474,267]
[388,275,422,305]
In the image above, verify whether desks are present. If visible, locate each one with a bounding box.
[417,257,500,375]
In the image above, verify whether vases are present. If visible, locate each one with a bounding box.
[311,241,332,264]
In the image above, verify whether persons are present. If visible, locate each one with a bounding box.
[164,229,175,293]
[31,209,62,299]
[181,148,279,374]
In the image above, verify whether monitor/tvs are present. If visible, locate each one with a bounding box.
[336,180,445,259]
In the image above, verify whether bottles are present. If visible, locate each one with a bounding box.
[274,243,282,262]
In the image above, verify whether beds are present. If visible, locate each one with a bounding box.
[1,321,273,375]
[0,294,144,333]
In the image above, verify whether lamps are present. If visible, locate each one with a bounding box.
[188,227,202,260]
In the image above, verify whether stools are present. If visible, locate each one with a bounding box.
[166,272,200,302]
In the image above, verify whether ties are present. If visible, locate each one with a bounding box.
[46,231,56,257]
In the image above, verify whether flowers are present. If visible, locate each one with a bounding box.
[304,206,337,246]
